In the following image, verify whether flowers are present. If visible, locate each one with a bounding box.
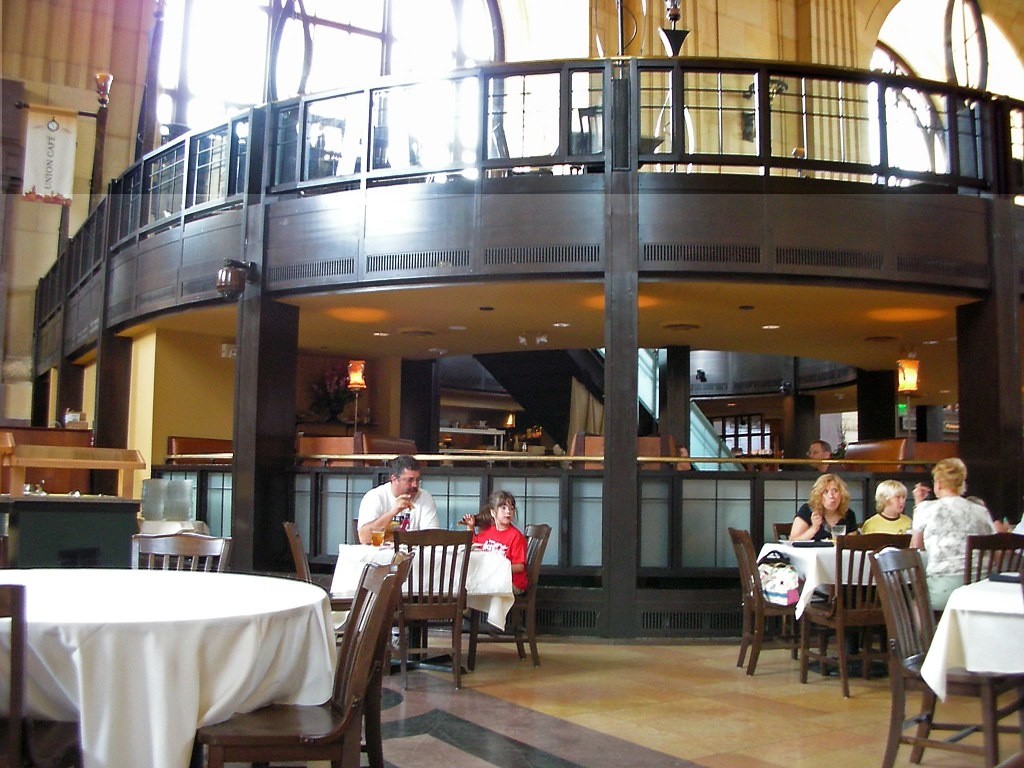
[305,363,362,416]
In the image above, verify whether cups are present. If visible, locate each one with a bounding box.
[831,525,846,547]
[370,528,384,546]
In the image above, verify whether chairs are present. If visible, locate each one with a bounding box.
[462,524,553,672]
[774,523,828,659]
[578,107,605,174]
[393,528,474,692]
[727,527,829,676]
[0,583,79,768]
[283,521,356,647]
[491,123,555,176]
[964,532,1024,586]
[867,546,1024,768]
[130,521,234,572]
[800,533,912,700]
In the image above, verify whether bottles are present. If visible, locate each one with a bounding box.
[504,425,543,452]
[1003,517,1008,525]
[747,449,779,472]
[28,479,47,491]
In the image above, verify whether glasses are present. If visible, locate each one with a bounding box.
[806,450,824,455]
[399,477,423,483]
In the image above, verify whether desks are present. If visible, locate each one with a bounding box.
[330,544,515,674]
[0,570,337,768]
[296,432,416,468]
[742,543,1024,704]
[553,131,665,171]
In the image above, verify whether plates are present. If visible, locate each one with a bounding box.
[821,539,833,542]
[779,540,815,546]
[24,491,47,497]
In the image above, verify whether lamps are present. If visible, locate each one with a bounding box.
[779,380,791,396]
[696,370,707,382]
[346,360,366,434]
[663,0,681,30]
[897,359,920,438]
[93,72,114,107]
[221,344,237,360]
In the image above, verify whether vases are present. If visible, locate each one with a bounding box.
[323,408,344,425]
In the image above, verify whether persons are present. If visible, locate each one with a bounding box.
[807,440,832,472]
[789,473,859,542]
[456,490,528,619]
[357,455,439,545]
[729,447,747,470]
[754,455,774,472]
[861,479,912,534]
[670,446,698,470]
[909,457,1024,642]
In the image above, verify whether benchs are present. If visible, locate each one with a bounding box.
[842,438,959,472]
[565,432,604,470]
[167,436,233,465]
[660,433,677,471]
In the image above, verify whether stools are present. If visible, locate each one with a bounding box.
[196,551,417,768]
